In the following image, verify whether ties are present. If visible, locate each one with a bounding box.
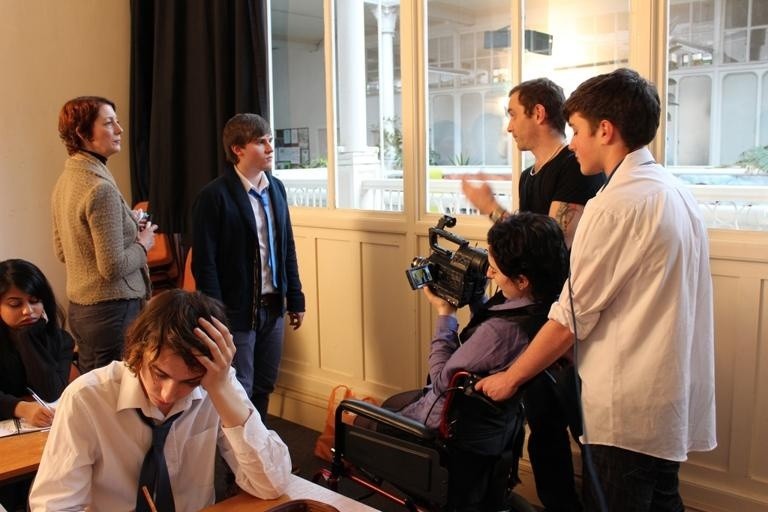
[133,406,186,511]
[248,187,280,288]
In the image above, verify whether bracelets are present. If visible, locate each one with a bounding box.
[488,207,506,224]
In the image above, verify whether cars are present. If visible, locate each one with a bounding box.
[656,159,767,230]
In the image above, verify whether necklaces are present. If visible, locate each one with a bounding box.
[529,144,561,177]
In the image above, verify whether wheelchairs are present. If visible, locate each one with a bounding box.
[331,364,540,512]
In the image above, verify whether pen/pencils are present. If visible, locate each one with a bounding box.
[25,387,50,410]
[141,485,158,512]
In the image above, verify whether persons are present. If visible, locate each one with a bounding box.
[49,93,159,376]
[472,68,717,512]
[352,207,572,433]
[23,286,294,512]
[190,112,307,497]
[0,258,76,431]
[459,74,584,511]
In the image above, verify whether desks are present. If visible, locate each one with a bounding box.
[202,474,382,512]
[0,428,48,481]
